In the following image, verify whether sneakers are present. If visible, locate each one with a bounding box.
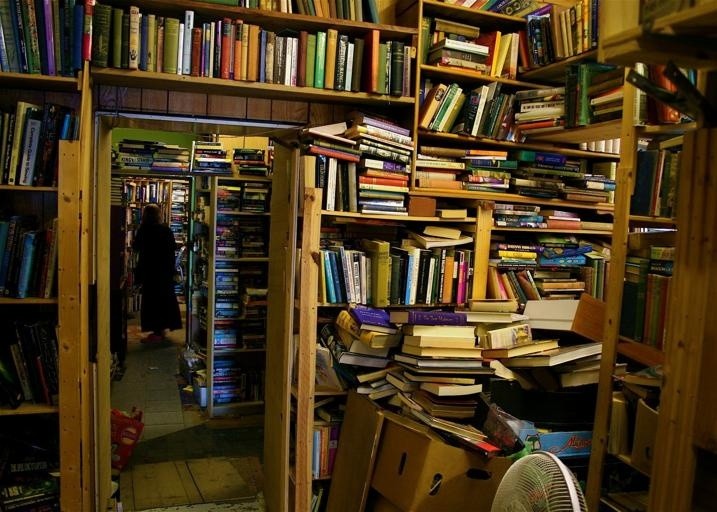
[140,333,165,343]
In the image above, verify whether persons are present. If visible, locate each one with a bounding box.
[131,204,182,344]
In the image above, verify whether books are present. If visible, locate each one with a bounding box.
[144,14,176,240]
[176,0,717,512]
[1,1,144,512]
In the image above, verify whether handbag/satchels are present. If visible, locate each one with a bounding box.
[111,407,144,476]
